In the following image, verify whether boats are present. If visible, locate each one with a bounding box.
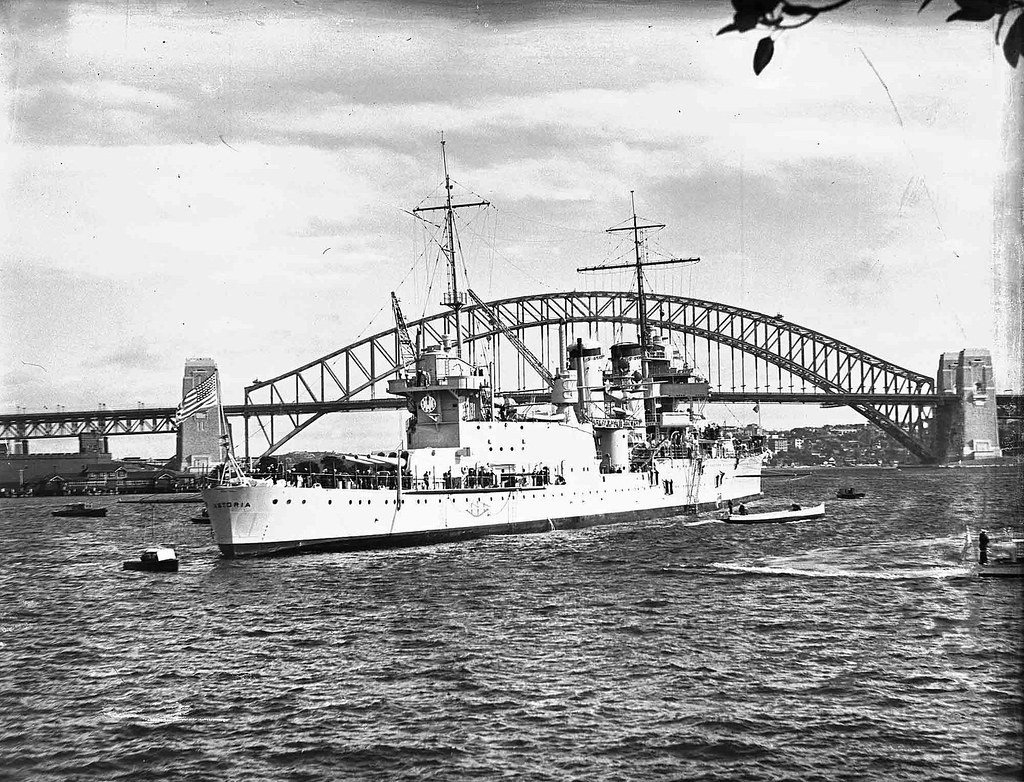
[717,501,826,524]
[956,525,1024,576]
[122,547,180,573]
[835,488,865,499]
[191,507,211,524]
[853,460,899,469]
[50,502,107,517]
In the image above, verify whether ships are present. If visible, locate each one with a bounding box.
[200,124,763,565]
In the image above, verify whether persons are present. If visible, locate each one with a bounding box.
[206,459,563,495]
[744,506,750,515]
[598,428,766,481]
[738,502,745,515]
[977,529,991,567]
[728,498,734,515]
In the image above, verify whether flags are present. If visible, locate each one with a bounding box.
[752,404,759,413]
